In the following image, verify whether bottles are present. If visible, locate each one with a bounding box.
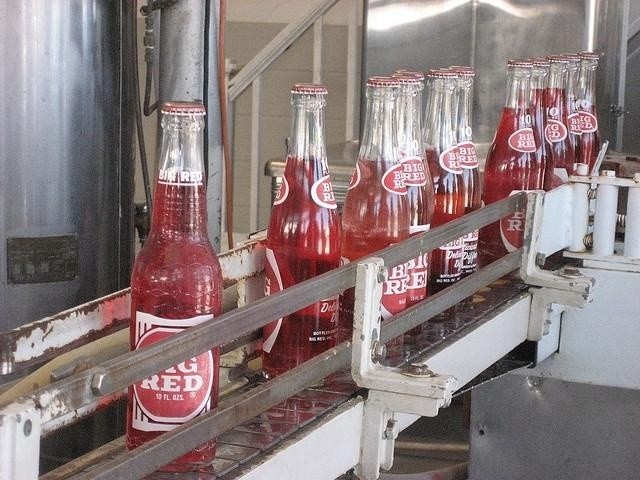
[261,83,342,390]
[125,101,223,470]
[341,51,603,358]
